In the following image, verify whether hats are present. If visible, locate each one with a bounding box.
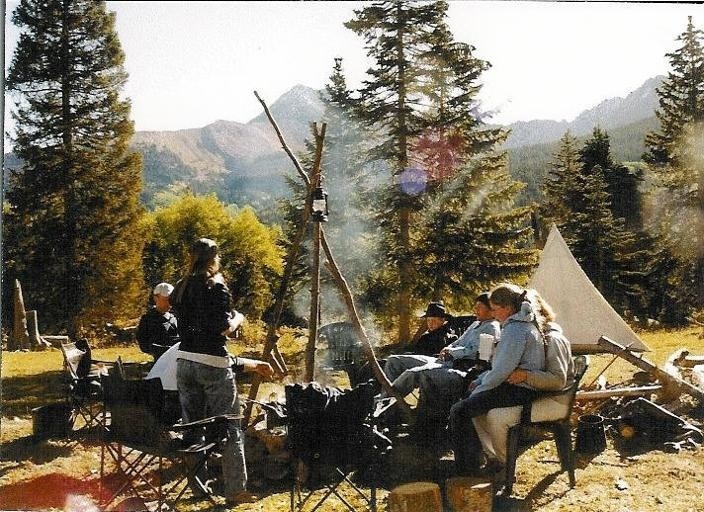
[153,282,174,297]
[419,303,453,318]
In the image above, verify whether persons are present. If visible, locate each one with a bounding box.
[381,284,573,478]
[168,238,261,503]
[137,283,180,361]
[146,341,274,427]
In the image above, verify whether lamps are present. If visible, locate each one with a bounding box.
[309,170,329,223]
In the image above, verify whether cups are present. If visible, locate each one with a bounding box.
[479,333,494,361]
[228,329,238,339]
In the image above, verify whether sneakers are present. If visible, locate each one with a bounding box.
[225,492,258,503]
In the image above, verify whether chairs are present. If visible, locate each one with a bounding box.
[60,339,131,439]
[317,322,366,371]
[284,386,377,511]
[503,354,591,496]
[100,372,244,512]
